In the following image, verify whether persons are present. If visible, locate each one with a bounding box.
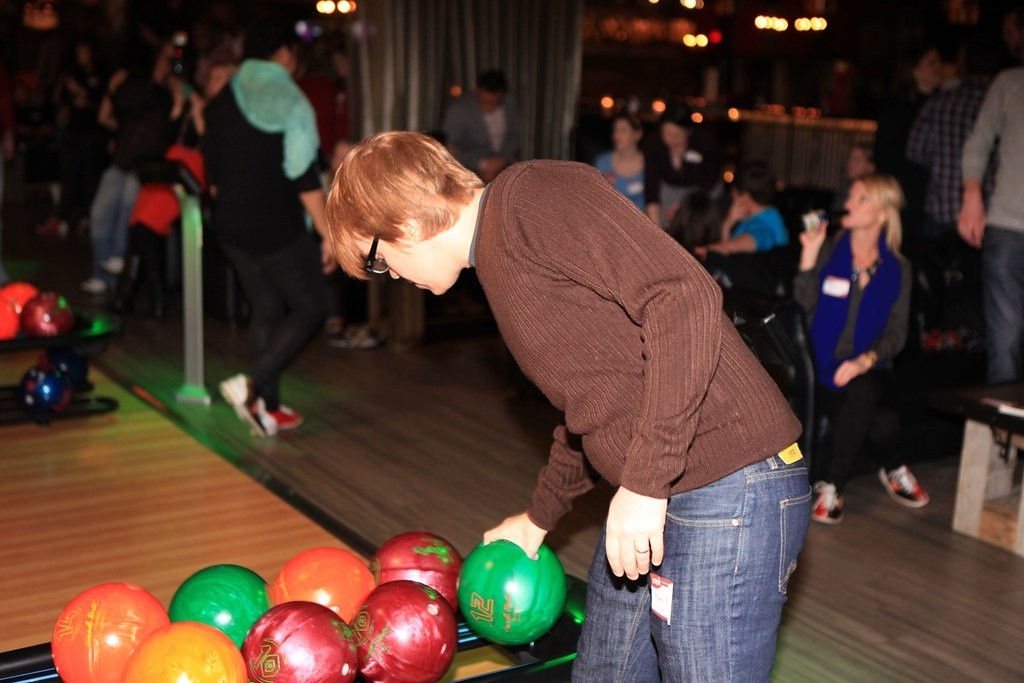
[590,106,791,306]
[427,71,520,318]
[798,13,1024,524]
[326,131,812,683]
[0,0,391,439]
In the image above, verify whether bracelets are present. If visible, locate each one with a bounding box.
[865,350,877,368]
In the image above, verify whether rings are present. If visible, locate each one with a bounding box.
[635,547,650,554]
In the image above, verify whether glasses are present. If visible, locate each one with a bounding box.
[363,235,391,274]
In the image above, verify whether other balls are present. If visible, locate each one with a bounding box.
[368,530,461,613]
[1,281,72,339]
[168,563,270,648]
[458,540,568,647]
[242,599,354,683]
[16,362,74,420]
[118,621,247,682]
[272,546,376,626]
[351,580,458,683]
[50,583,168,682]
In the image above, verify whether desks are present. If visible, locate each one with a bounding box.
[947,382,1023,557]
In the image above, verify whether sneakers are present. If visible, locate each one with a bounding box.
[879,464,928,507]
[811,480,846,525]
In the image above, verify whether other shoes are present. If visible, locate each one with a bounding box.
[252,405,302,436]
[329,317,379,348]
[219,372,277,435]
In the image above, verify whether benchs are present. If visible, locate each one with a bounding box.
[712,237,993,487]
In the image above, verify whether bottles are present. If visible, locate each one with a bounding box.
[784,207,849,234]
